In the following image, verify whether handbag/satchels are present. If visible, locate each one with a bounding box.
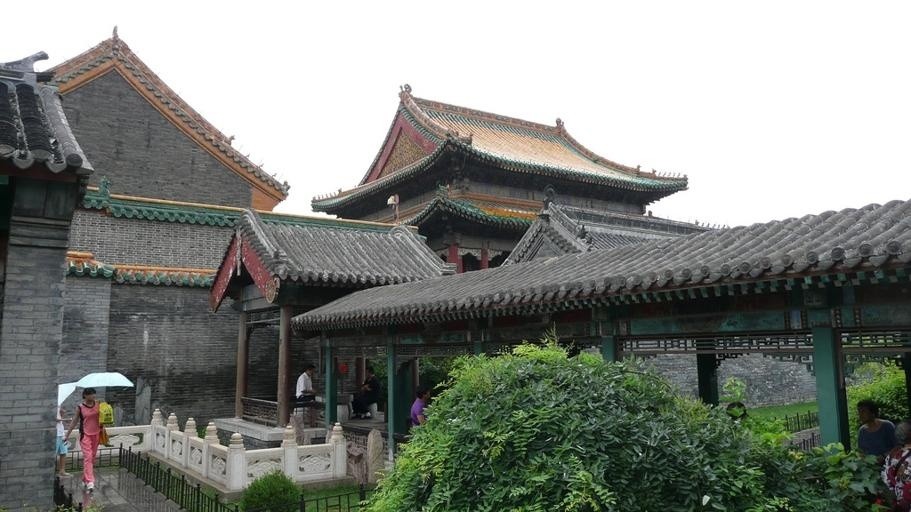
[98,402,115,426]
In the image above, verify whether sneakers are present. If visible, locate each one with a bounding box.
[55,468,94,495]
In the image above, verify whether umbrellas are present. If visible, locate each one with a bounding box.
[57,382,76,406]
[76,371,135,404]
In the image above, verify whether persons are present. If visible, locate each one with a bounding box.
[872,420,911,512]
[855,399,899,466]
[358,366,380,420]
[55,405,72,477]
[63,388,105,494]
[295,363,326,412]
[350,367,372,414]
[410,385,432,428]
